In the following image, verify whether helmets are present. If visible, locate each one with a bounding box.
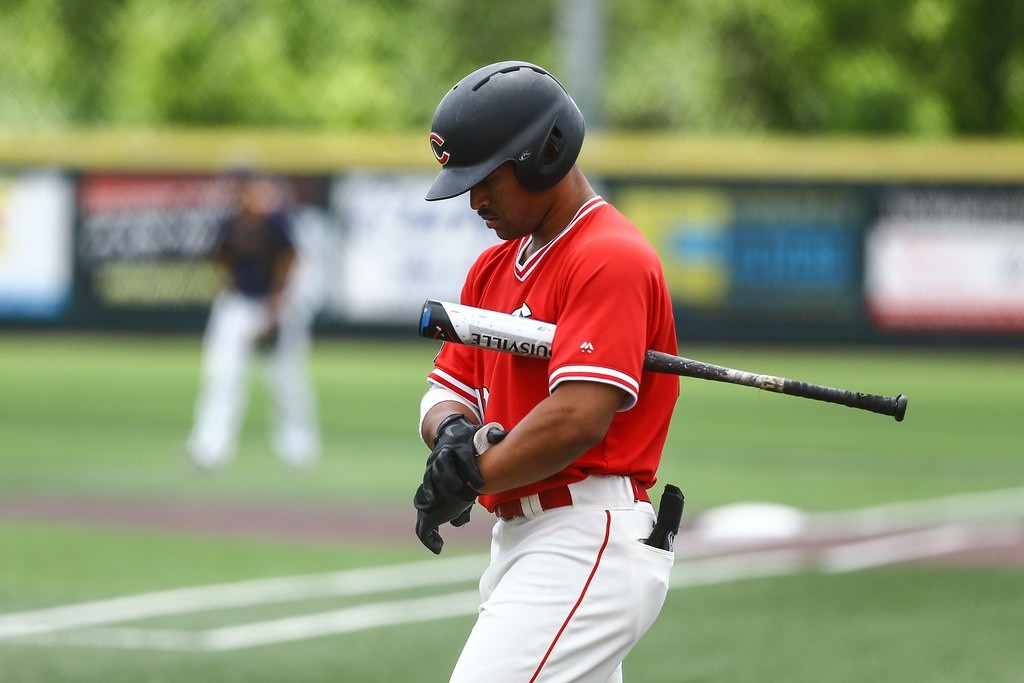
[425,60,587,202]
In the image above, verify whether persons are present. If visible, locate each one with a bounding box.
[186,174,322,466]
[414,61,681,681]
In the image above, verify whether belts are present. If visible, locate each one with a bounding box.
[494,477,651,521]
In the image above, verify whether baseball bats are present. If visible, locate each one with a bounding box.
[418,299,908,423]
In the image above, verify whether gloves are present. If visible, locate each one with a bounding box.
[415,481,482,556]
[413,414,512,509]
